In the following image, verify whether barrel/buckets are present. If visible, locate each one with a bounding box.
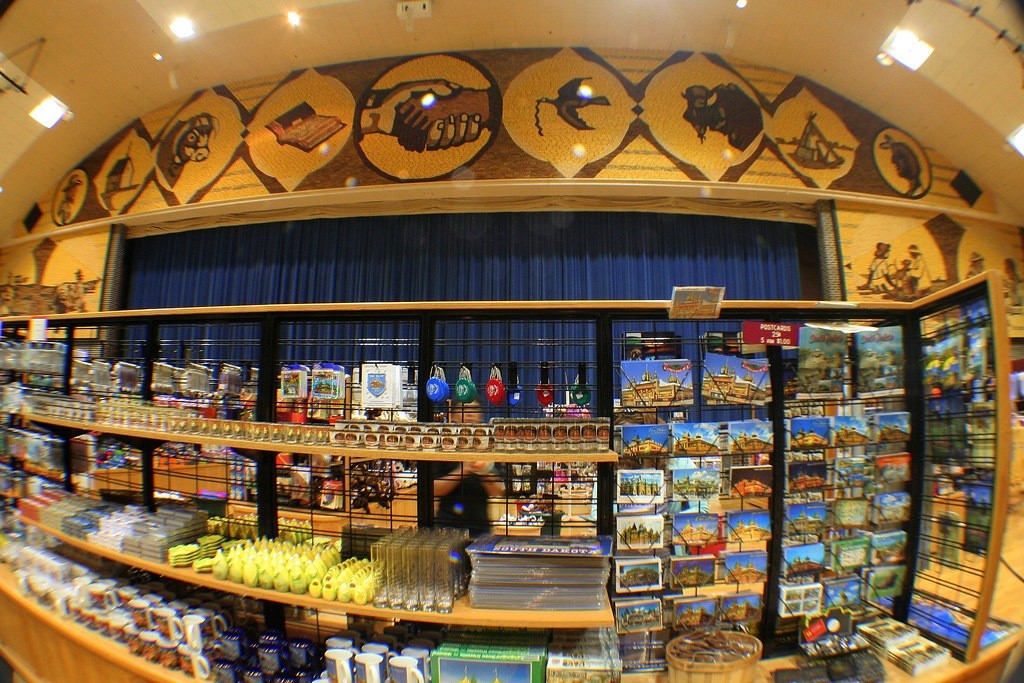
[667,631,763,683]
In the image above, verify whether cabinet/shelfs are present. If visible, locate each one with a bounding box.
[226,484,418,542]
[0,407,620,683]
[96,463,231,493]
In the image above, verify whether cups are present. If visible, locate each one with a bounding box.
[312,637,431,683]
[69,578,228,678]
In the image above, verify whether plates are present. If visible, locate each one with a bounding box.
[168,535,247,572]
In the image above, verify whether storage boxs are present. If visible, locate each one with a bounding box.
[431,623,624,683]
